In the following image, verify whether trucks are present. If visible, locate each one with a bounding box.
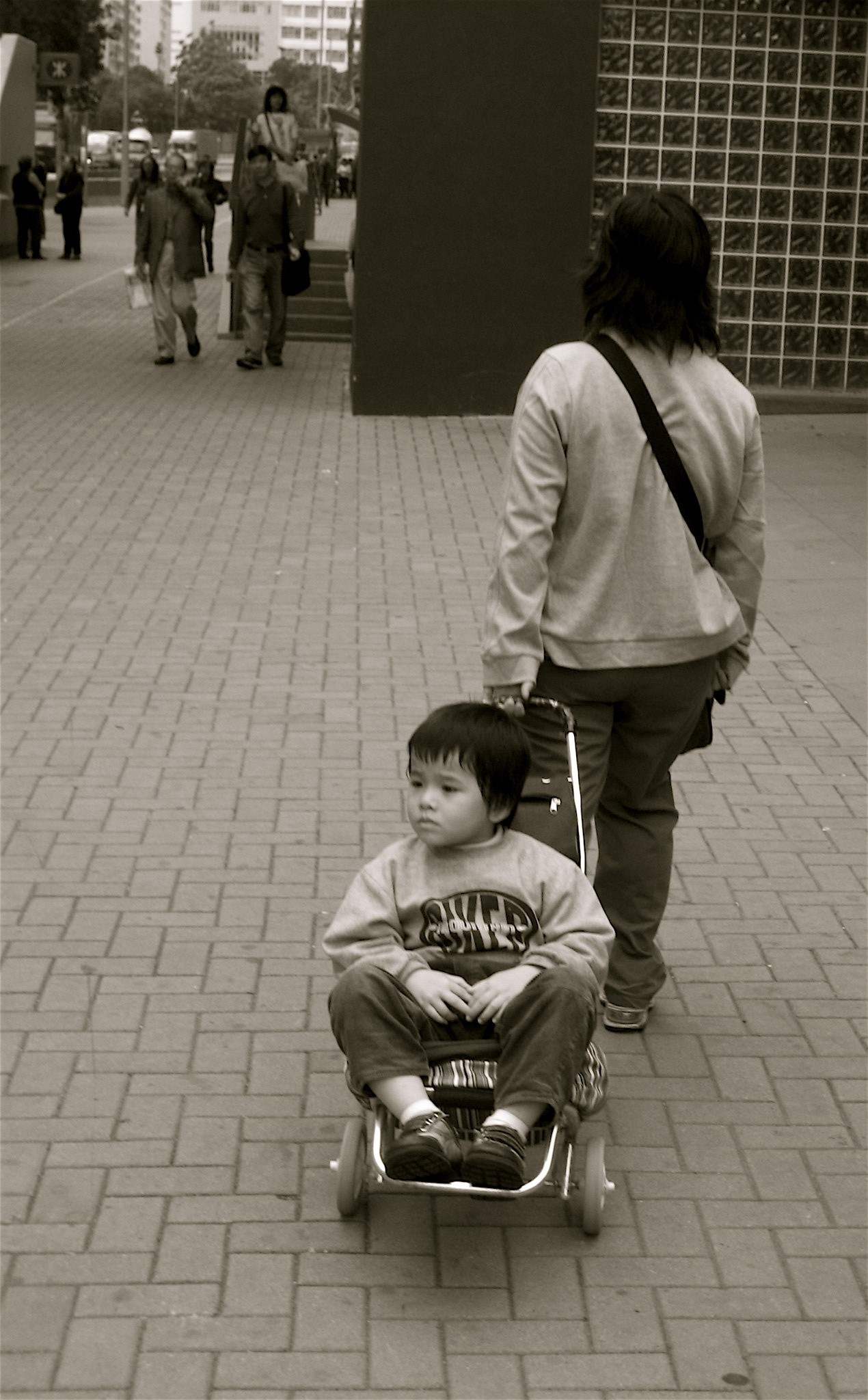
[163,128,220,170]
[33,100,58,164]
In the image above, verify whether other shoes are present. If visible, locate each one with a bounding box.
[602,1004,652,1028]
[32,252,43,259]
[58,254,70,259]
[269,357,283,366]
[54,202,61,213]
[187,336,200,356]
[73,254,80,261]
[237,357,262,369]
[209,264,214,273]
[155,356,174,364]
[19,253,29,259]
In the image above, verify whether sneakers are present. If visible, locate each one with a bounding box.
[464,1125,526,1202]
[385,1111,462,1184]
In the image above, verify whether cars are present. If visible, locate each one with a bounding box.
[87,129,121,170]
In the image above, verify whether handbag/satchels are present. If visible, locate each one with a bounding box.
[681,698,713,755]
[125,263,153,310]
[282,250,311,296]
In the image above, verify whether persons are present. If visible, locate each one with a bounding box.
[124,155,165,282]
[225,146,299,369]
[135,153,213,366]
[322,704,615,1186]
[185,155,228,277]
[252,85,302,167]
[483,190,765,1032]
[176,149,356,207]
[12,156,47,260]
[57,155,85,260]
[36,150,48,240]
